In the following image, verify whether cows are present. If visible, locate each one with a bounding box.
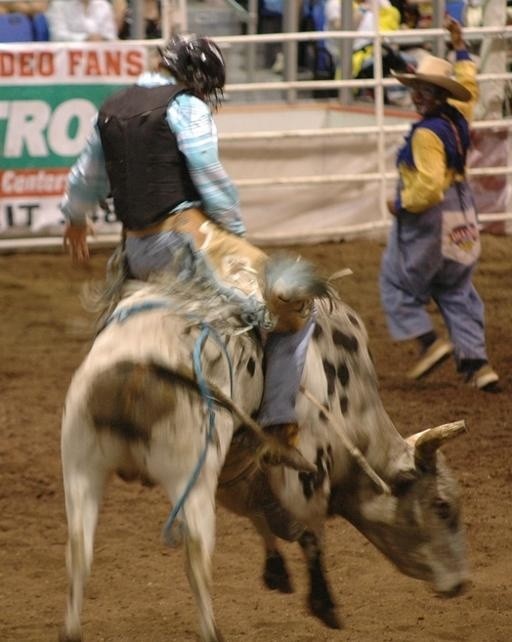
[54,263,476,642]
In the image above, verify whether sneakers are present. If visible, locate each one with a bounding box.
[262,438,319,473]
[463,365,499,388]
[405,337,453,381]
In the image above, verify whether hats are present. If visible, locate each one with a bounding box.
[393,57,474,102]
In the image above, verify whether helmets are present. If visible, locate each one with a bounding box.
[166,35,226,97]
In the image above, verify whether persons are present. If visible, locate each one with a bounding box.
[376,11,502,390]
[56,32,318,477]
[2,0,162,42]
[237,0,512,106]
